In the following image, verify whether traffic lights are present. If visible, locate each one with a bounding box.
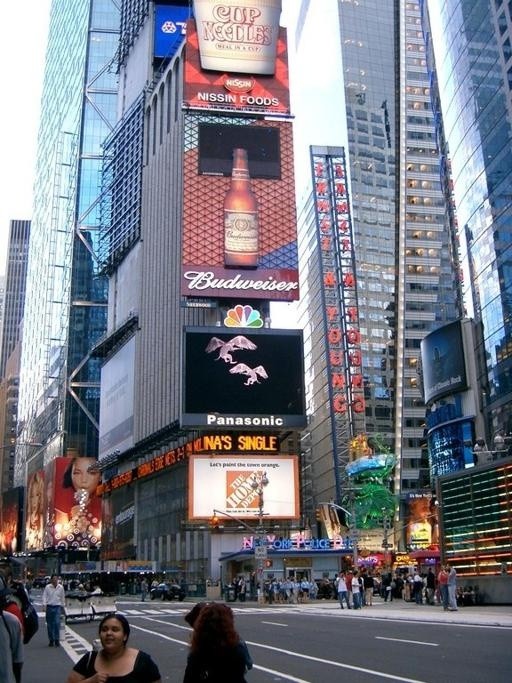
[213,515,220,534]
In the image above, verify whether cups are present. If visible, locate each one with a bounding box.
[194,1,282,74]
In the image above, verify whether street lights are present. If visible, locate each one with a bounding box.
[251,472,269,604]
[0,442,43,449]
[318,484,358,572]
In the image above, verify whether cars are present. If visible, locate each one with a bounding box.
[149,581,186,601]
[33,577,47,587]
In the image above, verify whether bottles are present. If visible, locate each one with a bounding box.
[224,148,259,267]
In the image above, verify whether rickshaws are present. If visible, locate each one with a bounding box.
[65,586,117,622]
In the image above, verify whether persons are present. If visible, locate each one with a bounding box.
[54,457,101,544]
[42,573,66,646]
[25,473,44,550]
[331,564,374,609]
[183,602,254,683]
[0,573,38,683]
[67,614,162,683]
[232,576,319,604]
[374,562,458,610]
[141,577,159,602]
[463,584,480,603]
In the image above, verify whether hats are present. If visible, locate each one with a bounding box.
[0,576,17,597]
